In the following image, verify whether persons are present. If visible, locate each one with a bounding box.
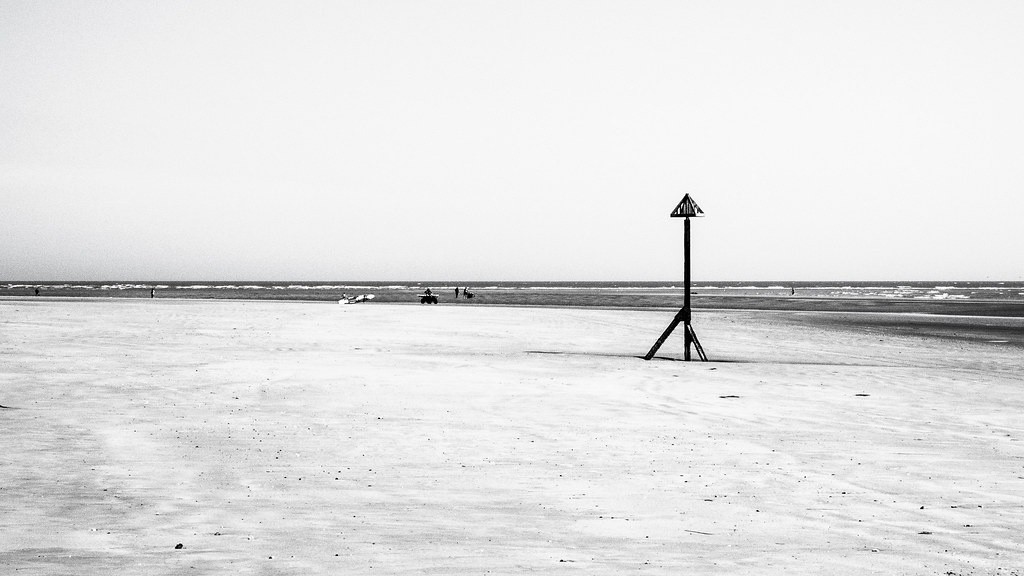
[341,292,347,299]
[454,286,460,298]
[463,286,469,300]
